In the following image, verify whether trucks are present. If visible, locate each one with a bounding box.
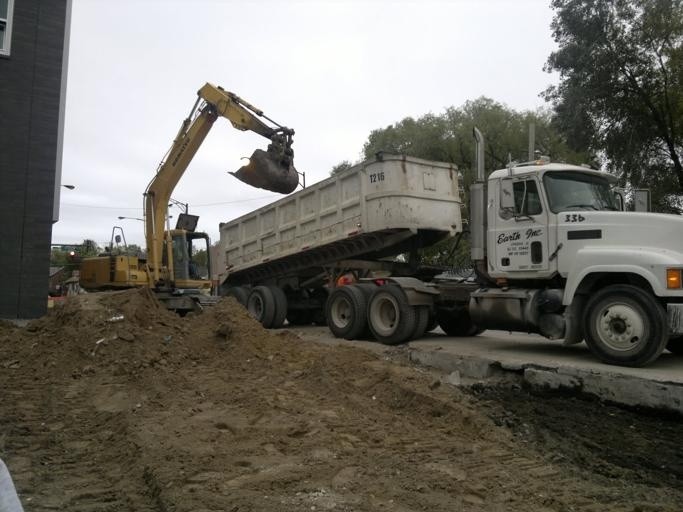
[214,121,683,367]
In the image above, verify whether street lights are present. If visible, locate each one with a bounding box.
[116,216,144,221]
[69,251,74,268]
[59,185,74,191]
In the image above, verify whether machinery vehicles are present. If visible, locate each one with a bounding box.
[73,79,298,309]
